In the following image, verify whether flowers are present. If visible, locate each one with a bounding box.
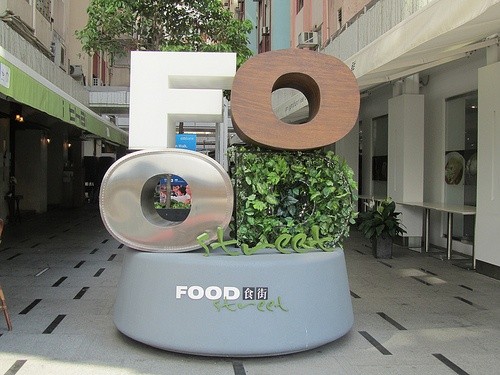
[358,196,407,239]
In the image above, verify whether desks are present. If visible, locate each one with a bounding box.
[356,195,387,213]
[395,201,477,269]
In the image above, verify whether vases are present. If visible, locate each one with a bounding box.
[373,232,393,259]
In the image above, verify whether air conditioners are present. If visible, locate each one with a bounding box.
[262,26,270,35]
[93,78,103,86]
[69,65,82,75]
[297,32,318,48]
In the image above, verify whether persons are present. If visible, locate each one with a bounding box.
[160,184,192,207]
